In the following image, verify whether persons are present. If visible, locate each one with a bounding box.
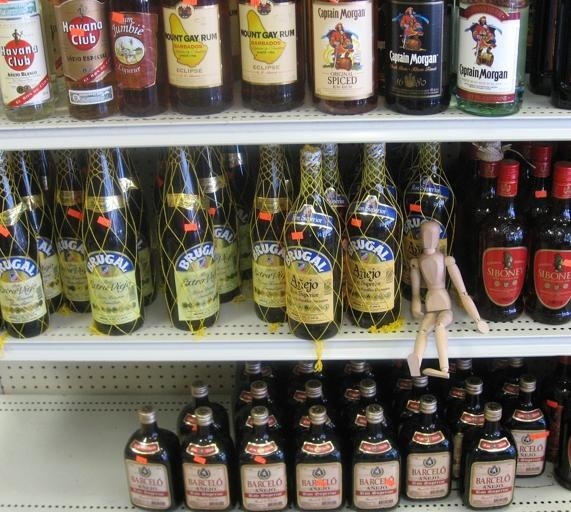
[329,22,354,58]
[472,15,496,56]
[400,7,424,39]
[405,221,490,379]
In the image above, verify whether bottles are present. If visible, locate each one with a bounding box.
[0,144,571,339]
[0,0,570,124]
[123,357,571,511]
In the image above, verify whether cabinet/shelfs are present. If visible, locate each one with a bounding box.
[0,84,571,511]
